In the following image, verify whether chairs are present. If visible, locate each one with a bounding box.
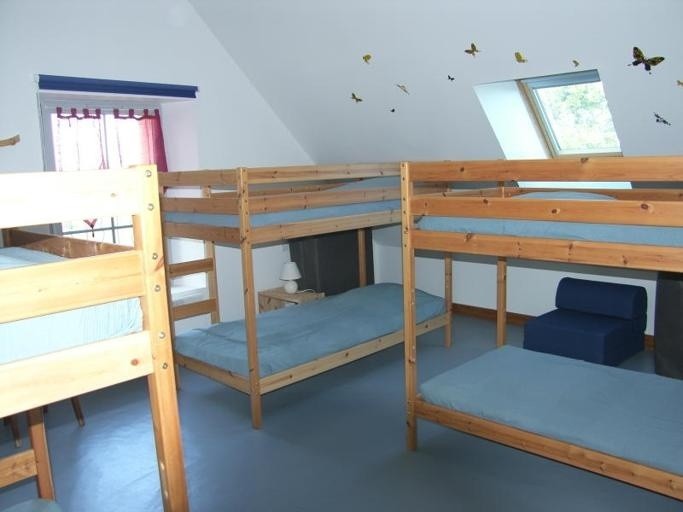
[524,277,647,366]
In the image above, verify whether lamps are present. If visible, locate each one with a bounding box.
[279,262,301,295]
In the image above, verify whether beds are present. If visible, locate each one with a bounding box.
[157,159,451,428]
[0,164,189,512]
[398,155,683,501]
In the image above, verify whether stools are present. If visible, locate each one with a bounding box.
[4,396,87,447]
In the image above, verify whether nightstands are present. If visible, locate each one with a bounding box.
[258,288,324,313]
